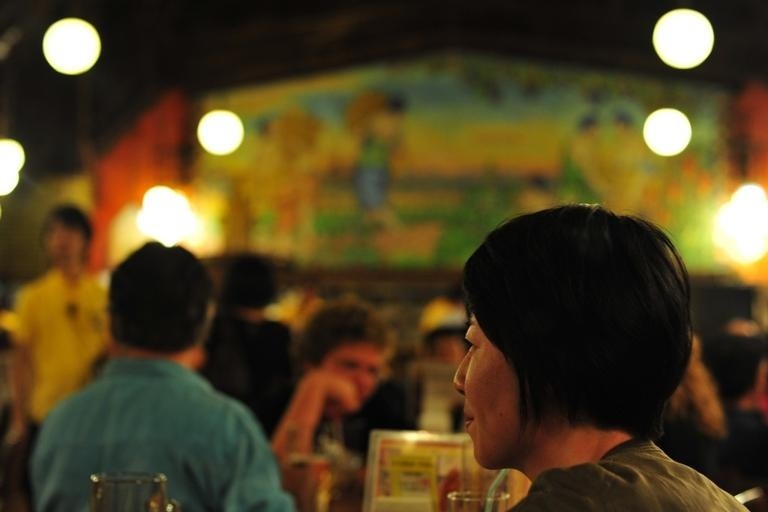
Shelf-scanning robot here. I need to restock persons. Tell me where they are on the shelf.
[265,300,406,467]
[670,327,732,438]
[200,252,298,440]
[29,242,297,512]
[689,314,767,491]
[454,201,750,511]
[6,201,110,445]
[422,293,470,362]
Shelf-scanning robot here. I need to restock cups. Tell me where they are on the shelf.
[447,491,510,512]
[287,453,332,512]
[89,471,168,511]
